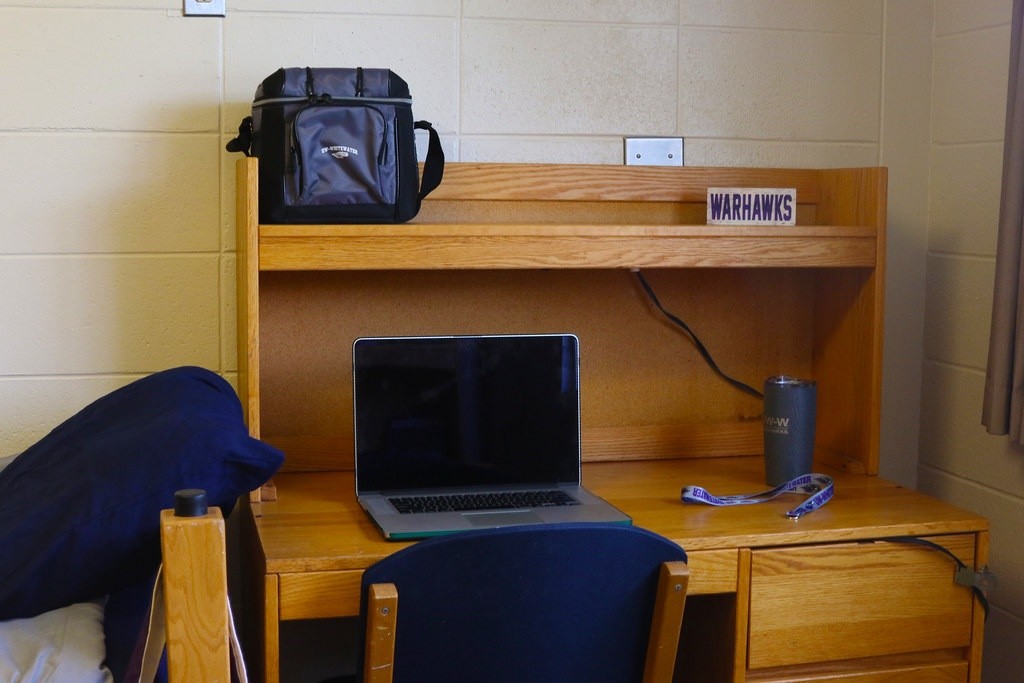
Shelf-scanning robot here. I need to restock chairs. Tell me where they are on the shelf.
[357,522,691,683]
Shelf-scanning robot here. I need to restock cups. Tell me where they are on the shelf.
[765,379,815,487]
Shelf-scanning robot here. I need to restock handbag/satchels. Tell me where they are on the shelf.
[226,67,445,224]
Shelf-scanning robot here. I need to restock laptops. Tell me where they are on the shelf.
[351,332,633,541]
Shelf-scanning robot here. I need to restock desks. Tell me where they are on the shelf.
[230,162,990,683]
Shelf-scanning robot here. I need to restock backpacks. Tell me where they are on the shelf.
[0,366,284,623]
